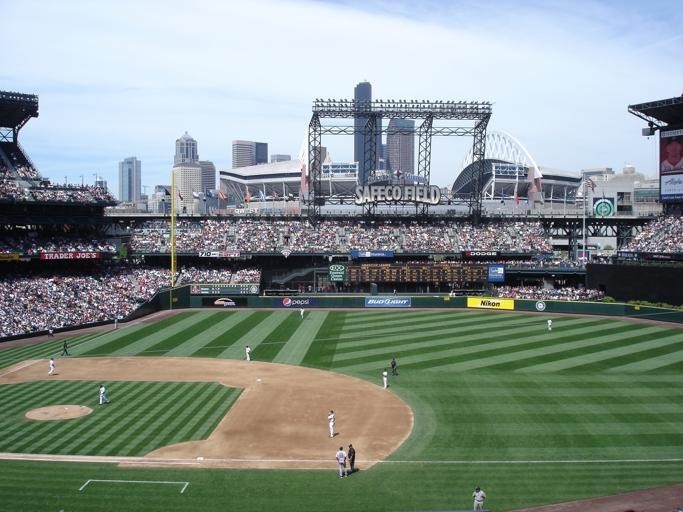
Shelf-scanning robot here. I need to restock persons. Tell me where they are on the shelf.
[98,385,110,405]
[659,137,682,171]
[244,345,251,361]
[381,368,388,389]
[48,357,56,376]
[335,446,347,479]
[327,410,335,437]
[390,358,397,376]
[471,486,486,512]
[347,444,356,472]
[60,339,70,357]
[0,152,682,340]
